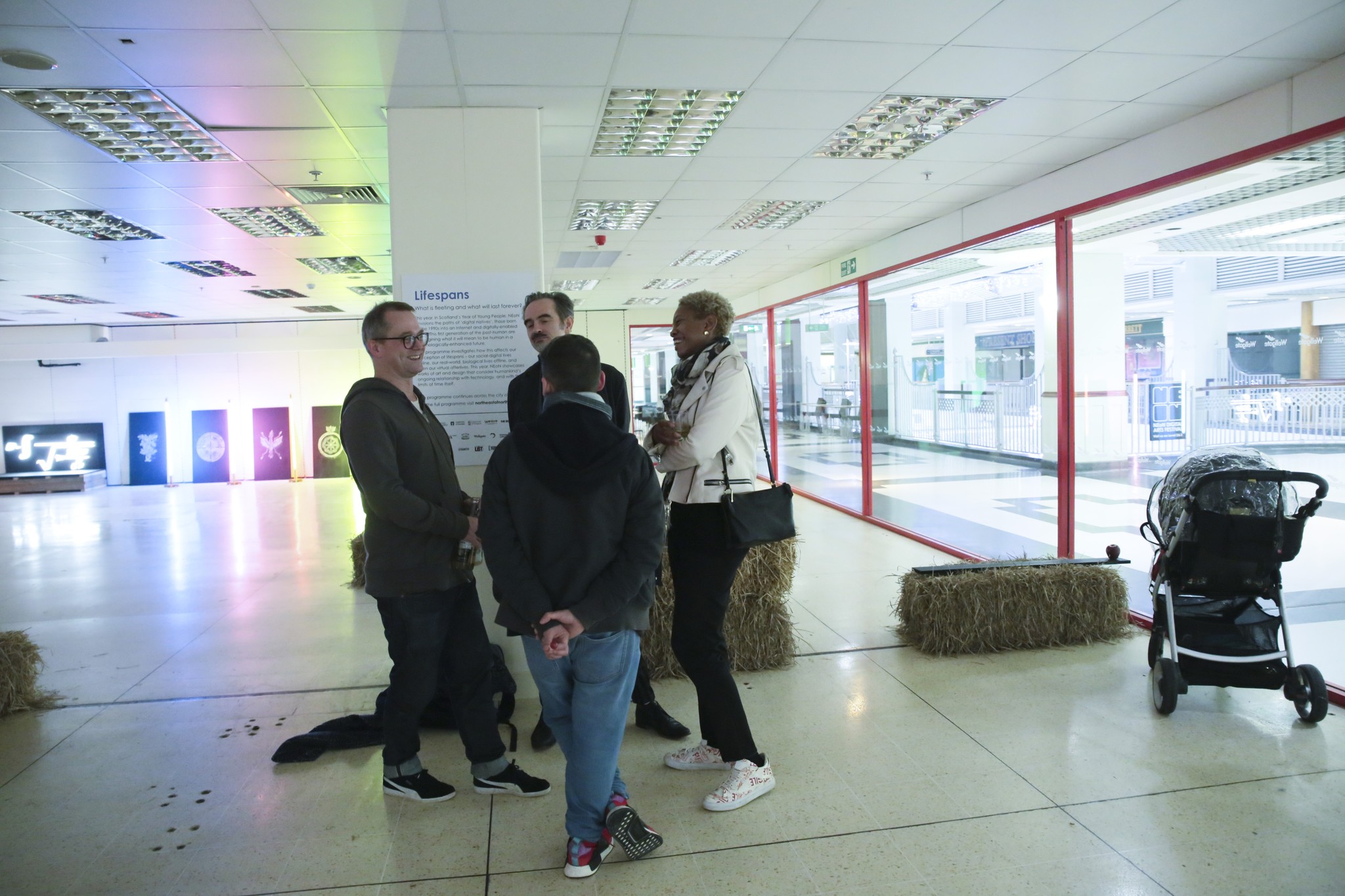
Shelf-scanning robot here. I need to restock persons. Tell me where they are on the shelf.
[644,289,777,812]
[474,334,664,879]
[507,291,691,753]
[341,302,552,803]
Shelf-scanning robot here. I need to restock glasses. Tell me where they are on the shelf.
[373,332,429,349]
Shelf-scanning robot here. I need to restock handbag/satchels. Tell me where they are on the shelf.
[720,480,796,549]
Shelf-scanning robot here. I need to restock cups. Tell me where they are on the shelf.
[663,409,691,441]
[453,497,483,571]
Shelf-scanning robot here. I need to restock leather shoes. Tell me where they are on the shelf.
[531,707,557,750]
[635,700,691,739]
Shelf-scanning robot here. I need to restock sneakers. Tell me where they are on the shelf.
[702,750,776,810]
[473,761,551,798]
[382,753,457,802]
[663,739,732,771]
[604,795,663,861]
[563,827,614,878]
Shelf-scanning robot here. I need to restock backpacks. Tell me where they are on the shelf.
[419,642,519,753]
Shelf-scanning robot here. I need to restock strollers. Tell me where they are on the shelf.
[1141,448,1327,721]
